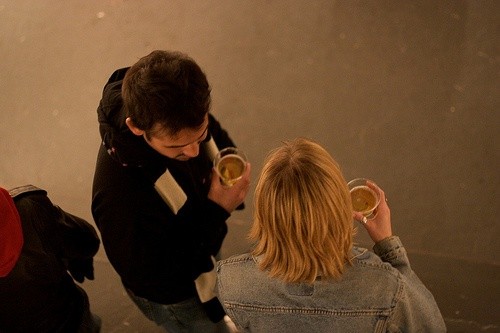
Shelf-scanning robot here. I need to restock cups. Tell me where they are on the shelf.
[214,148,248,186]
[347,178,381,218]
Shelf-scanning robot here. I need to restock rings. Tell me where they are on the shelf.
[360,216,368,225]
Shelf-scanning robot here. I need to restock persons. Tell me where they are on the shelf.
[0,184,101,333]
[90,49,250,333]
[216,137,450,333]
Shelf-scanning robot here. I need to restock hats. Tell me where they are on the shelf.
[0,187,24,279]
